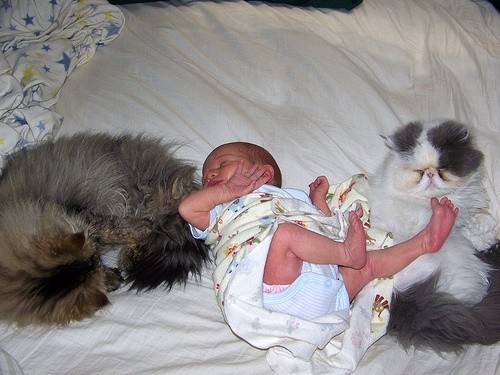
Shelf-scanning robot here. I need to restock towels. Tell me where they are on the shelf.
[202,173,396,375]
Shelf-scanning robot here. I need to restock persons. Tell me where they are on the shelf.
[178,141,459,362]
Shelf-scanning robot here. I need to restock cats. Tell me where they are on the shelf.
[1,128,213,331]
[374,118,500,361]
